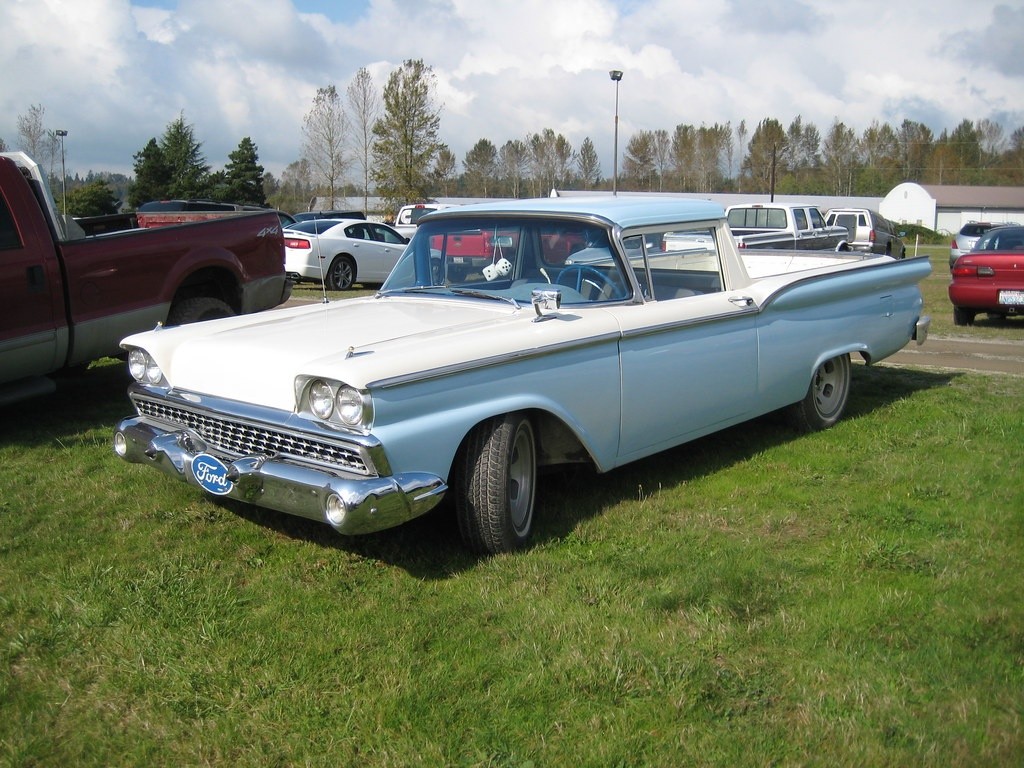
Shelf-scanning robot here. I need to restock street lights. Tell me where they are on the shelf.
[55,129,70,239]
[610,69,624,194]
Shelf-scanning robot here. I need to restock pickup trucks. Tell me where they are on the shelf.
[663,203,850,257]
[0,151,293,392]
[111,196,932,555]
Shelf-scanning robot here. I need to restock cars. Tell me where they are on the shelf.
[395,203,462,239]
[428,224,588,270]
[282,217,411,289]
[565,228,664,267]
[951,222,1002,269]
[949,223,1024,326]
[138,201,299,232]
[281,211,376,241]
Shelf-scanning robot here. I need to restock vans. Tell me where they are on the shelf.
[823,207,906,260]
